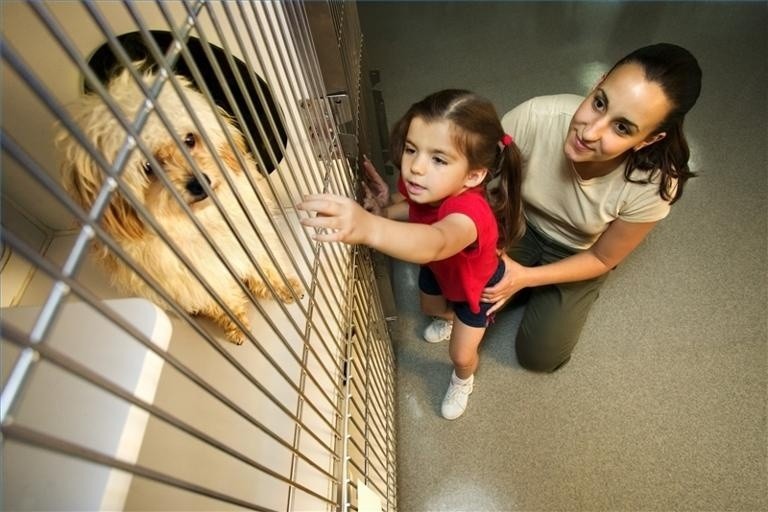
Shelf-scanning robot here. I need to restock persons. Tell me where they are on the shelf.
[295,89,523,422]
[478,40,704,376]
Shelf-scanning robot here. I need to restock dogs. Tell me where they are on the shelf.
[51,60,306,346]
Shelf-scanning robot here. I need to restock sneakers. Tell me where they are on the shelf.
[423,317,453,344]
[440,368,474,421]
